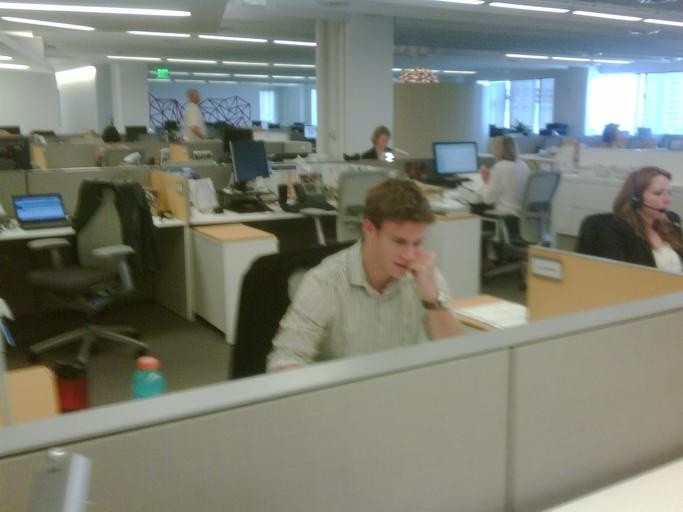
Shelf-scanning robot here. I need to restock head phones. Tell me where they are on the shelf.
[629,174,642,208]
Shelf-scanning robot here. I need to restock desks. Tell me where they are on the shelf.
[2,121,470,242]
[1,246,681,512]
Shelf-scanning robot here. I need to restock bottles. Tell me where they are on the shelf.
[50,356,89,415]
[127,354,164,402]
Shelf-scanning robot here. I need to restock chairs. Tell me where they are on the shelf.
[482,171,566,290]
[21,174,153,376]
[297,164,400,257]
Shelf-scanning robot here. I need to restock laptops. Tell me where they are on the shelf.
[10,193,73,231]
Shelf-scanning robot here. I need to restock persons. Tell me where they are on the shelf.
[183,89,206,142]
[95,126,130,166]
[594,167,683,275]
[472,136,529,261]
[361,125,393,159]
[265,179,462,374]
[601,124,623,148]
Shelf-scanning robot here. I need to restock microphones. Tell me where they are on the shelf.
[643,204,666,213]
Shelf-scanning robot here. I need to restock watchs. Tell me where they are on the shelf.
[421,289,449,310]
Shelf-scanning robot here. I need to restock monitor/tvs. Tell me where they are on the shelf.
[125,126,146,138]
[303,125,317,140]
[165,119,177,129]
[0,134,32,169]
[432,141,478,176]
[229,140,269,186]
[223,126,253,152]
